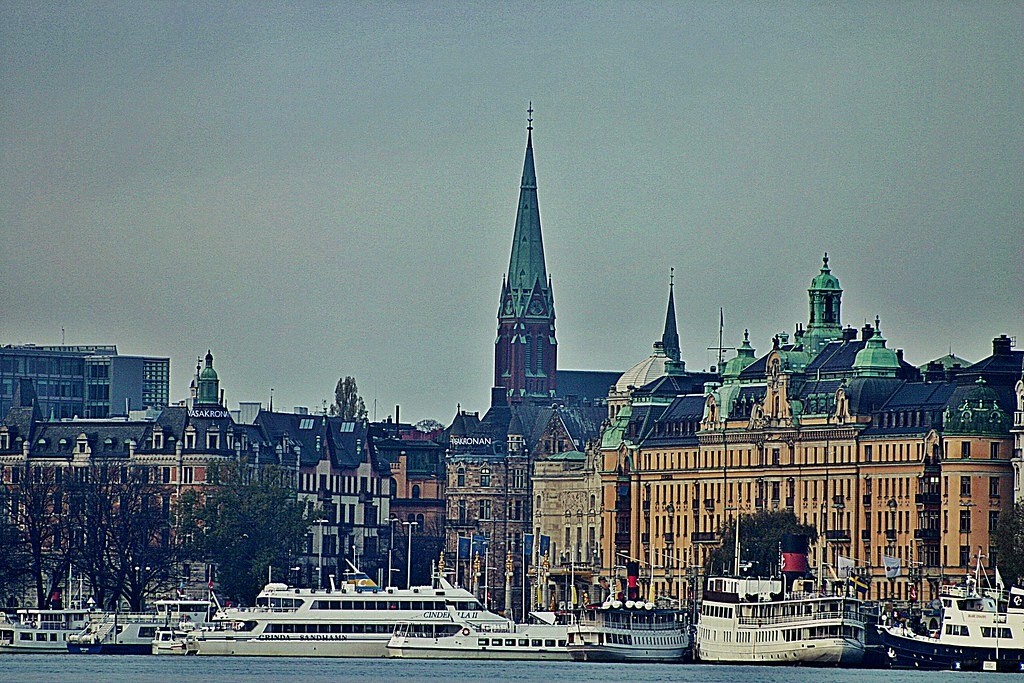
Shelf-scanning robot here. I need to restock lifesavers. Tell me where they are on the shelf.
[462,628,469,636]
[62,622,66,628]
[31,621,36,628]
[85,622,89,627]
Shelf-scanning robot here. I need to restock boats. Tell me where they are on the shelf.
[861,544,1024,675]
[694,491,870,669]
[0,563,110,655]
[66,593,247,656]
[563,544,696,664]
[386,530,580,662]
[151,549,515,660]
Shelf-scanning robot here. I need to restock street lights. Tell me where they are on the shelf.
[959,502,977,573]
[831,503,846,579]
[312,518,329,589]
[384,518,399,550]
[402,522,419,589]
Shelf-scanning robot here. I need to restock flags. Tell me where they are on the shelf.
[539,534,550,557]
[523,532,534,555]
[849,572,869,593]
[883,555,902,578]
[837,555,856,578]
[457,535,484,561]
[996,566,1005,590]
